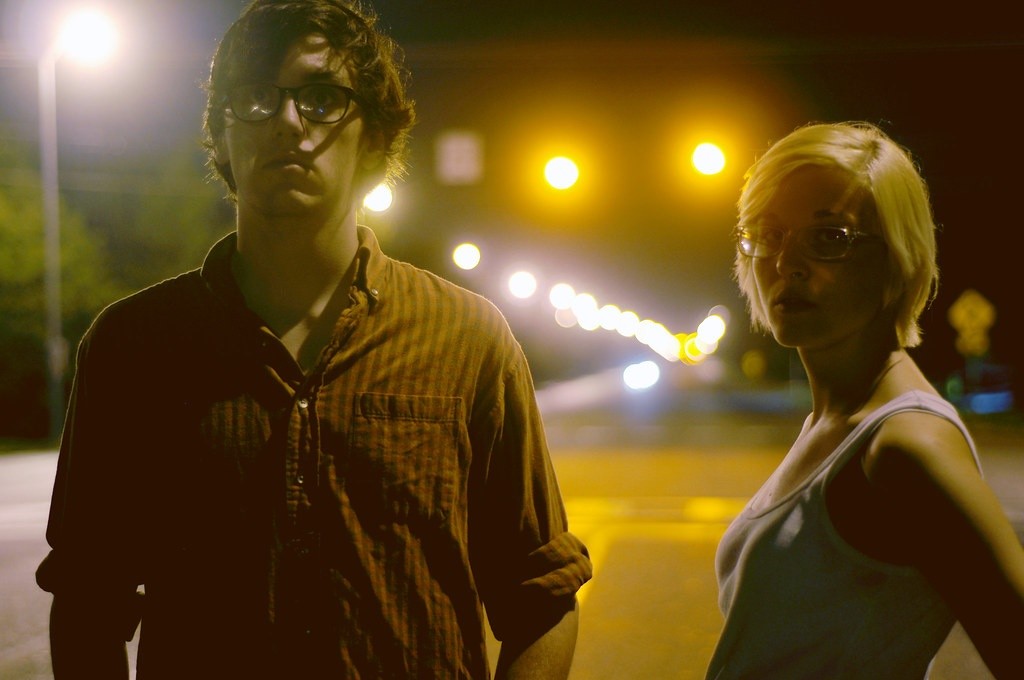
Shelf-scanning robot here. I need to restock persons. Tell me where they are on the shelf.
[705,122,1023,680]
[37,0,592,680]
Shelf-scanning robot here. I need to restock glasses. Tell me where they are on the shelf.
[735,223,886,259]
[218,79,373,123]
